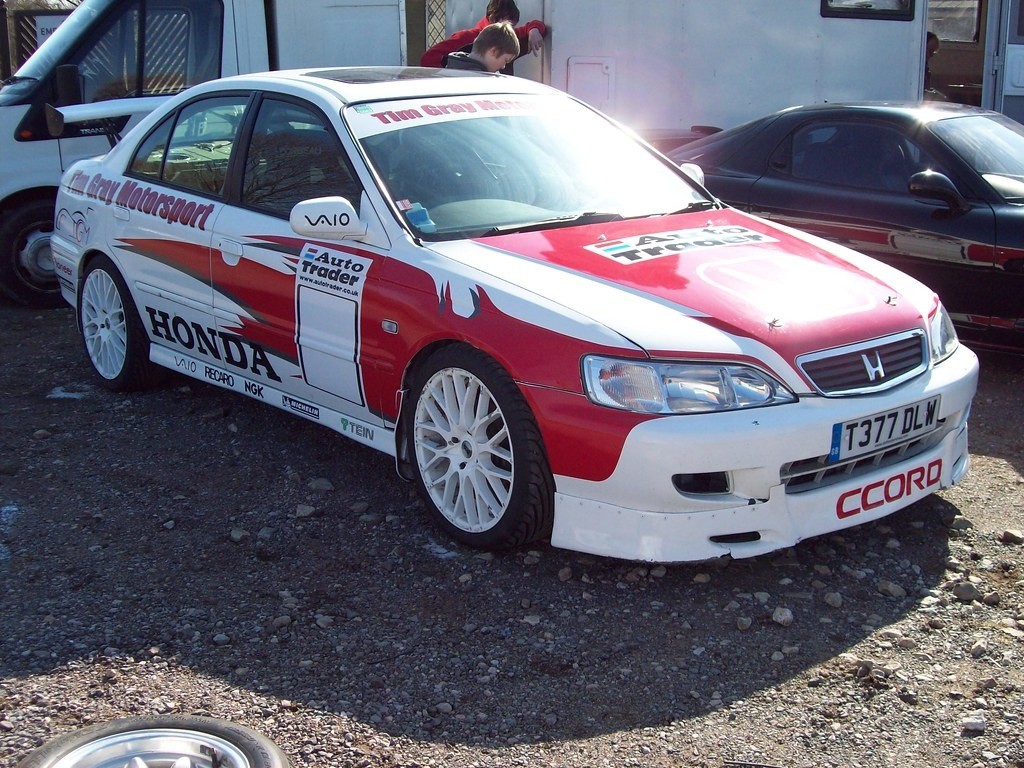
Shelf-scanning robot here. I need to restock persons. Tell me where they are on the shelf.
[445,21,520,75]
[923,30,944,102]
[419,0,546,78]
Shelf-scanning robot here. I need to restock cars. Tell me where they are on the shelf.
[658,97,1024,361]
[46,61,979,563]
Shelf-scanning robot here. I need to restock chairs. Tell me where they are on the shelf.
[257,129,358,207]
[877,140,913,193]
[396,148,486,200]
[805,143,850,183]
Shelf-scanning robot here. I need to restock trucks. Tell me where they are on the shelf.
[0,0,1024,317]
